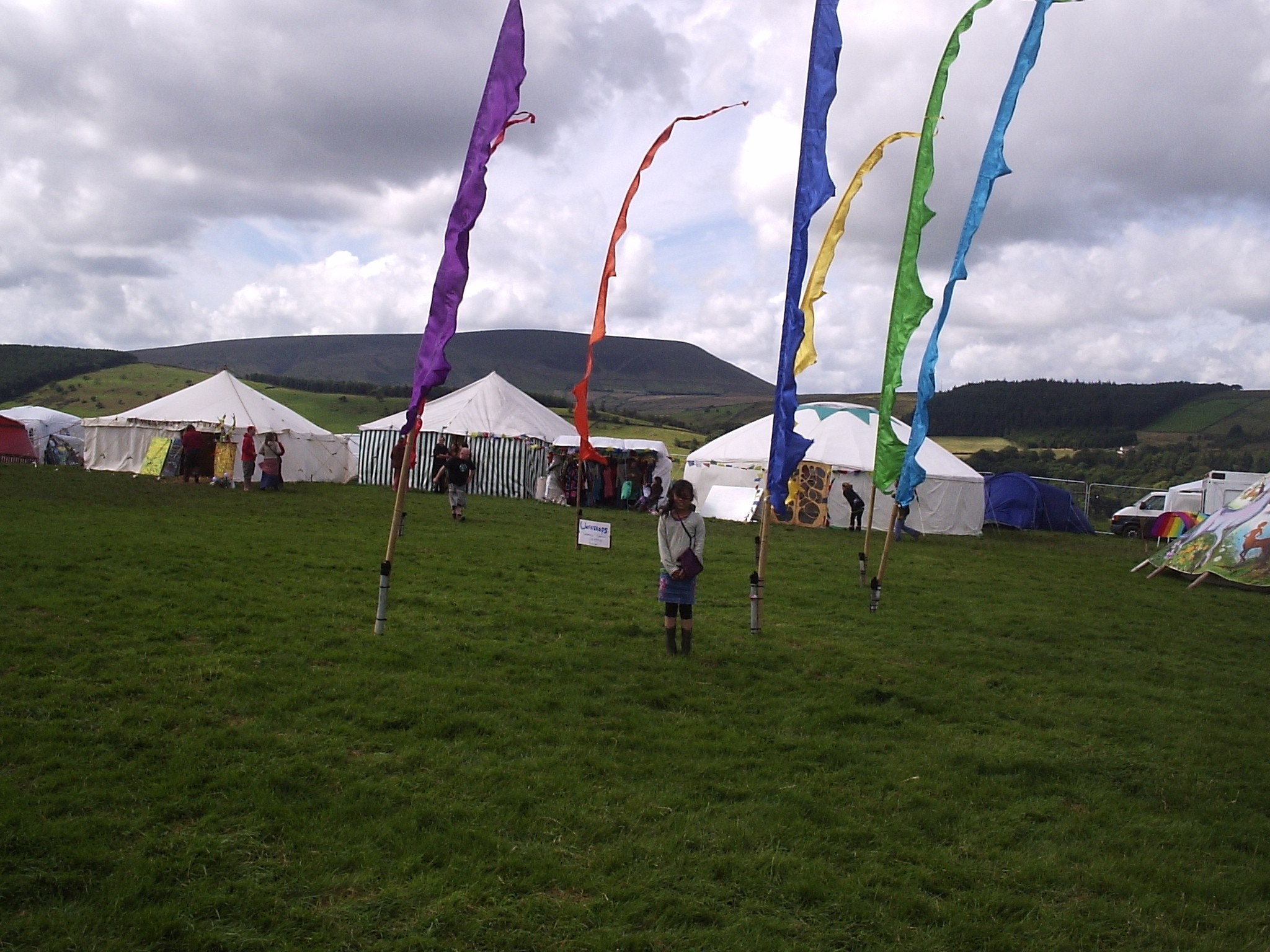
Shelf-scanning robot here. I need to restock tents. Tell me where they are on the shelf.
[985,473,1098,538]
[355,369,582,501]
[1131,470,1269,594]
[678,400,985,539]
[545,435,672,517]
[80,369,348,485]
[0,405,85,467]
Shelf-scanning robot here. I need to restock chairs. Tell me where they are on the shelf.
[645,497,664,513]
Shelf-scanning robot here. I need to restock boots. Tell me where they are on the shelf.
[628,501,644,512]
[665,626,678,655]
[681,626,693,654]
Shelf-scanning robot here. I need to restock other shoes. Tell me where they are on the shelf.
[913,533,923,543]
[244,487,253,492]
[849,526,855,531]
[856,526,862,531]
[451,510,466,522]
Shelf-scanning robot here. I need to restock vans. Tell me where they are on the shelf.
[1197,470,1270,519]
[1110,491,1208,543]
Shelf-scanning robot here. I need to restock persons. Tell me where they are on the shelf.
[627,460,641,491]
[241,426,258,491]
[657,479,705,658]
[840,482,865,531]
[258,432,286,490]
[627,476,663,512]
[180,425,205,485]
[433,436,472,493]
[432,448,475,521]
[893,478,921,543]
[390,437,405,491]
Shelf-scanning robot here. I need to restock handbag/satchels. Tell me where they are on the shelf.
[454,473,467,486]
[677,547,704,580]
[643,485,651,498]
[853,502,863,511]
